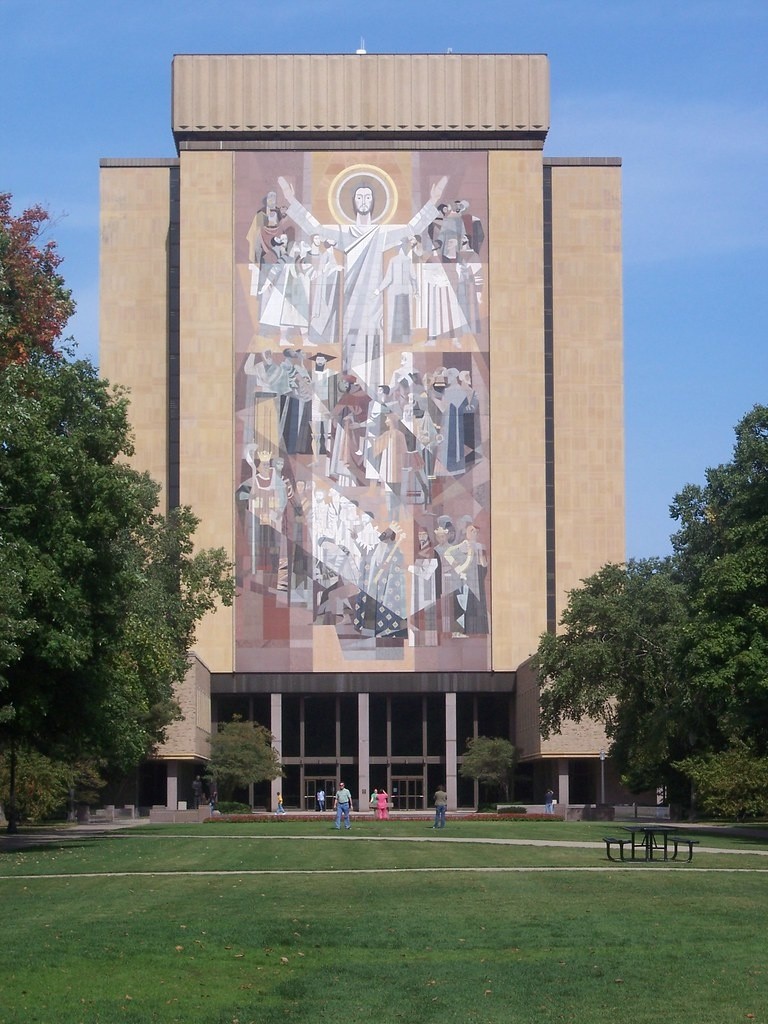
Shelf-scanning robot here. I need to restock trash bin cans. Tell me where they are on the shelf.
[589,804,617,821]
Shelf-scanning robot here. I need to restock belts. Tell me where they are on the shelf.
[338,802,348,804]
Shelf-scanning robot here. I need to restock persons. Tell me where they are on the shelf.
[376,789,388,820]
[317,787,326,812]
[370,789,378,811]
[192,776,201,809]
[274,792,286,815]
[334,782,353,829]
[433,785,448,828]
[545,788,555,813]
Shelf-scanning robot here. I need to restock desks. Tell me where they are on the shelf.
[625,827,679,862]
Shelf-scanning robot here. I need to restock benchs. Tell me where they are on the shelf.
[602,837,632,862]
[667,836,700,863]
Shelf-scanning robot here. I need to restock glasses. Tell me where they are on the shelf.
[340,785,344,786]
